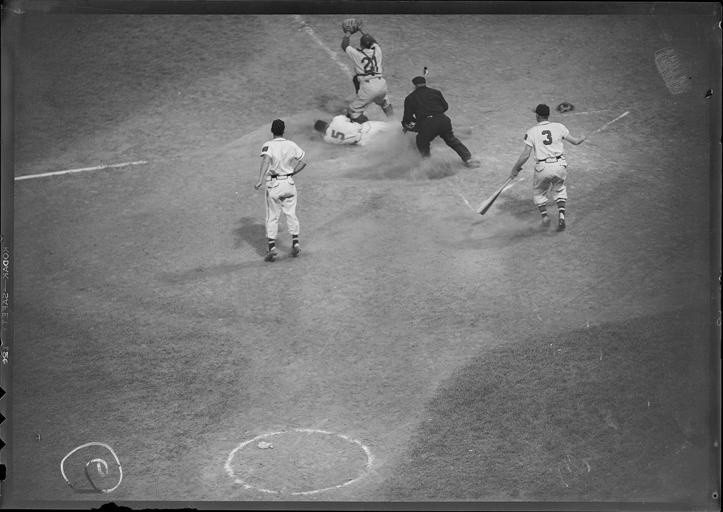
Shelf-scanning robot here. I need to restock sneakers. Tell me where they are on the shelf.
[466,159,480,167]
[559,213,565,230]
[264,248,280,262]
[293,243,301,257]
[544,217,550,228]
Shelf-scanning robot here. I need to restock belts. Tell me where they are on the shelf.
[538,156,560,162]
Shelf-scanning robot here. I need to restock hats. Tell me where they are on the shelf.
[535,104,550,115]
[272,174,294,178]
[271,119,284,134]
[412,76,426,85]
[314,120,328,131]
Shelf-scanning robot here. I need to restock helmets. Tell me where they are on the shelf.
[360,35,374,48]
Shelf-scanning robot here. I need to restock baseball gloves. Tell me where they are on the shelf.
[343,17,362,33]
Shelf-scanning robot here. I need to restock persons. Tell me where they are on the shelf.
[341,19,395,124]
[315,114,389,146]
[510,104,586,229]
[254,119,308,262]
[401,76,474,165]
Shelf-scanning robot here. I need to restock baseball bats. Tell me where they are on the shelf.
[480,176,512,214]
